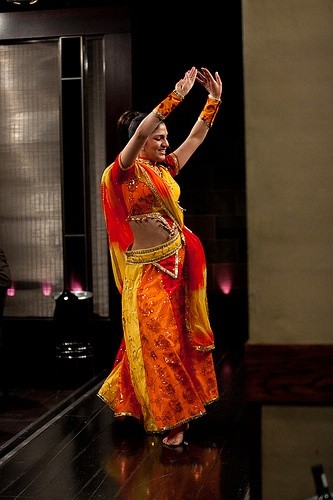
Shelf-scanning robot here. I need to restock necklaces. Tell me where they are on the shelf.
[139,159,173,192]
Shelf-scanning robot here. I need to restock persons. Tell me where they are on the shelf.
[96,65,223,446]
